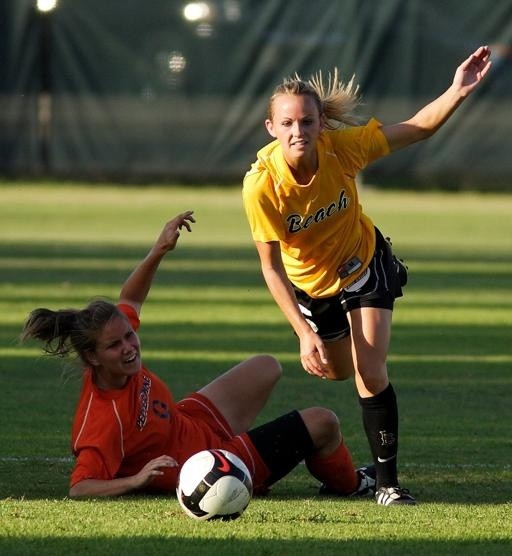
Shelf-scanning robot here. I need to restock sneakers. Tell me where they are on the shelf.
[318,465,376,497]
[375,483,417,506]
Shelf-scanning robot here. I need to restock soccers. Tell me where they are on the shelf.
[178,448,254,522]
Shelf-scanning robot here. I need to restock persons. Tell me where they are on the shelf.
[238,40,493,508]
[19,208,377,503]
